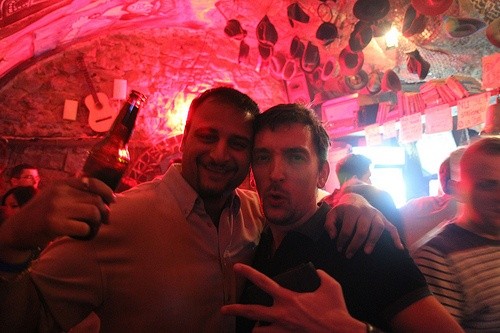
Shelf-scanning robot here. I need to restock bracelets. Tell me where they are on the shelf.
[366,323,374,333]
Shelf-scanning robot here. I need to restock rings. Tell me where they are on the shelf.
[81,176,90,189]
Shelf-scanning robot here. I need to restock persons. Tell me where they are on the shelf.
[219,101,464,333]
[0,87,406,333]
[0,163,44,280]
[316,153,463,252]
[397,138,500,333]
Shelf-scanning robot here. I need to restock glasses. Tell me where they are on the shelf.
[16,175,41,183]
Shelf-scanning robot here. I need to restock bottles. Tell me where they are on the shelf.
[68,90,147,240]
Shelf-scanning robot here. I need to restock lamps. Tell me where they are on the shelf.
[112,79,127,110]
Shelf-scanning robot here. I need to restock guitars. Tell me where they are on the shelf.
[75,50,117,133]
